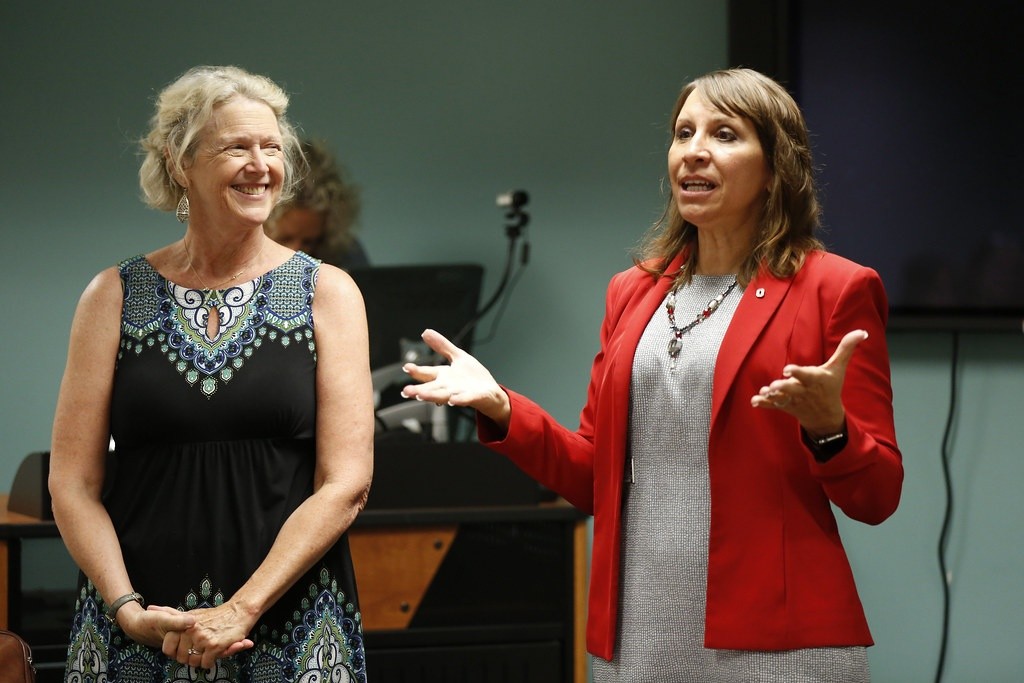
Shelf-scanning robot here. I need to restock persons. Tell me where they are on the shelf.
[264,141,374,312]
[402,67,906,683]
[47,66,376,683]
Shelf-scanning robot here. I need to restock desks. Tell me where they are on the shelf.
[0,492,590,683]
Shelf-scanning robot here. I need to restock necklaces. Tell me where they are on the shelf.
[666,261,740,358]
[183,236,267,291]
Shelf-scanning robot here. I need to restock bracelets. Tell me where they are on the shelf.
[105,592,144,623]
[801,421,847,448]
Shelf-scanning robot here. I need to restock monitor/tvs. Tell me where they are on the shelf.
[349,265,484,395]
[777,0,1024,330]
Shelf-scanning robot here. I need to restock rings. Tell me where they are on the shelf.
[188,647,201,655]
